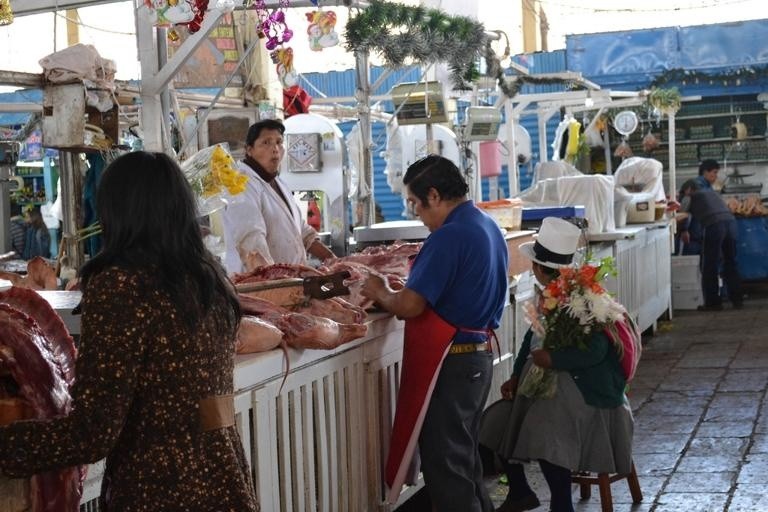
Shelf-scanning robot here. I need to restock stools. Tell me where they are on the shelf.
[570,456,643,511]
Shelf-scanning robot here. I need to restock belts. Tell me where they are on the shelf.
[449,341,488,354]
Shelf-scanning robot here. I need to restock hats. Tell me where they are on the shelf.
[680,179,704,195]
[518,217,580,270]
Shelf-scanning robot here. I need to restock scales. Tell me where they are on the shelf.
[723,108,756,177]
[462,106,541,227]
[521,205,586,230]
[614,110,638,163]
[353,82,450,241]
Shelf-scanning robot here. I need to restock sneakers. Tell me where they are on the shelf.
[697,300,744,310]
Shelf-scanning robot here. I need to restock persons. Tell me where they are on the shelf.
[0,151,260,511]
[360,152,510,512]
[221,120,339,278]
[476,217,642,511]
[675,159,749,301]
[678,180,745,311]
[22,206,51,259]
[9,199,26,257]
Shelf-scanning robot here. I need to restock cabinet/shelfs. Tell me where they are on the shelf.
[635,110,768,169]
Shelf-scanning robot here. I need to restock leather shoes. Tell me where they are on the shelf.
[495,494,540,511]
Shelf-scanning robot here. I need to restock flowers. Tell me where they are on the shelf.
[520,244,628,398]
